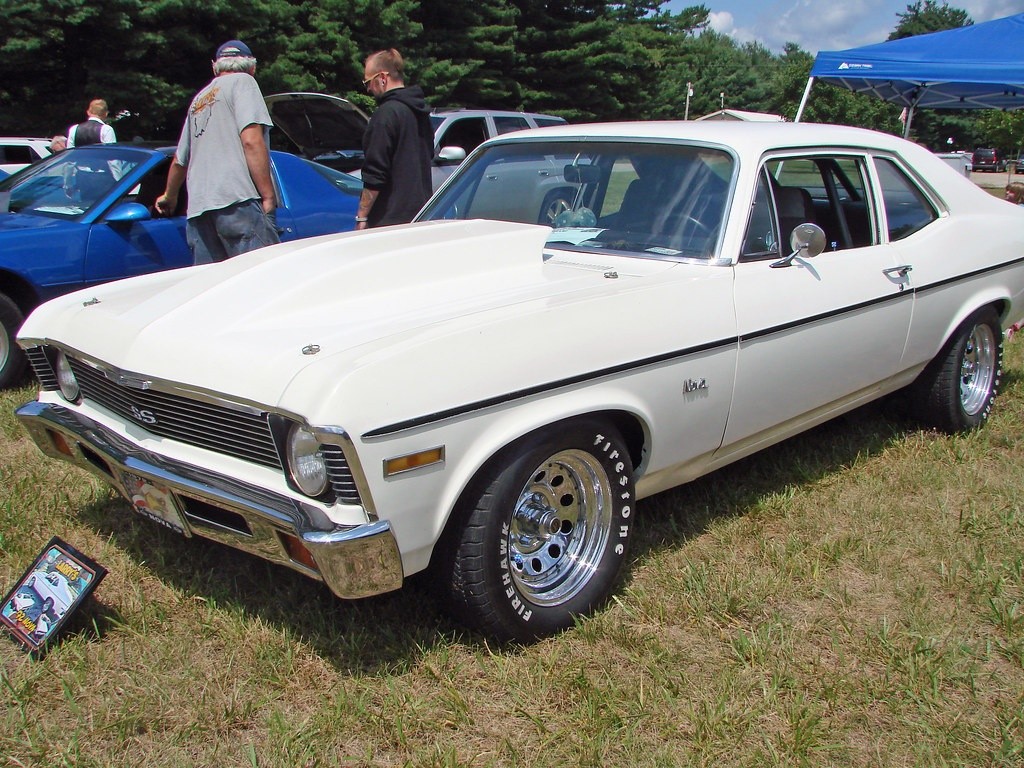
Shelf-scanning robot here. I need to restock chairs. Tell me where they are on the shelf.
[758,186,815,254]
[608,180,676,235]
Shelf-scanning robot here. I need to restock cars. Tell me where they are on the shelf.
[0,142,371,390]
[0,137,80,200]
[261,91,591,228]
[1015,154,1024,174]
[11,117,1024,651]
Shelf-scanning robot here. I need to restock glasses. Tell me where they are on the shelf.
[362,71,388,88]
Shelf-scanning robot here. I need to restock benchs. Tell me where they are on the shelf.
[682,192,923,249]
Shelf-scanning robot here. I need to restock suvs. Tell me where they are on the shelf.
[971,147,1004,175]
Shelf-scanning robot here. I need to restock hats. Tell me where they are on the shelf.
[216,40,252,58]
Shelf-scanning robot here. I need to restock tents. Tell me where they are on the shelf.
[769,13,1024,183]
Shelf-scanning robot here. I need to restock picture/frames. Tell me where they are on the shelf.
[0,536,109,661]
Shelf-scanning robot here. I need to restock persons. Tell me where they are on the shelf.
[62,100,123,205]
[154,40,277,266]
[353,47,439,231]
[49,136,69,154]
[586,145,739,262]
[1004,184,1023,207]
